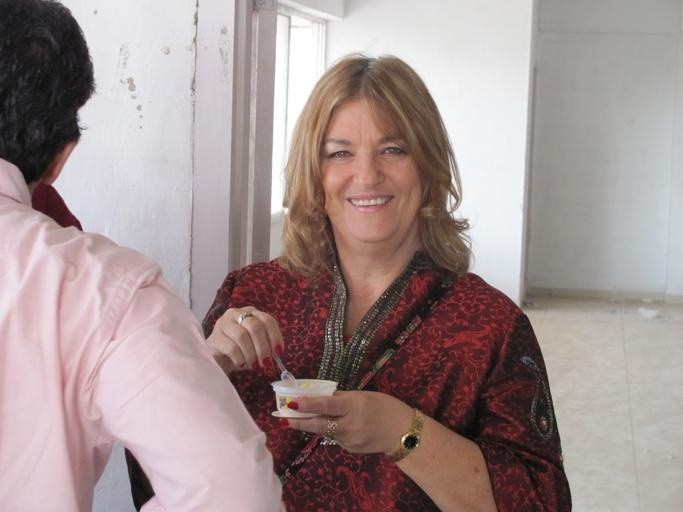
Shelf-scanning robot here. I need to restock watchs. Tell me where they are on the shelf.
[389,408,424,462]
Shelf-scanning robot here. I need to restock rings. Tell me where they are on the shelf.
[319,437,337,446]
[328,417,337,434]
[239,311,253,323]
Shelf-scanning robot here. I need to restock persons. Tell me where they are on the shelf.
[0,2,282,512]
[126,49,571,512]
